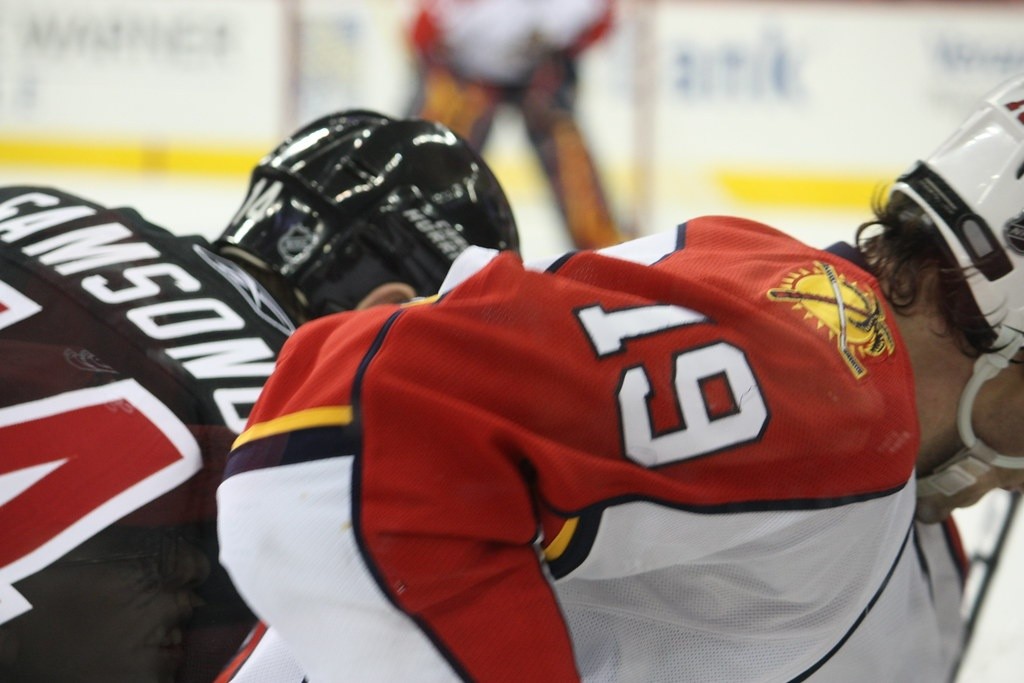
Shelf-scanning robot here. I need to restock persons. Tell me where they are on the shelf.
[390,2,621,253]
[215,73,1024,683]
[0,109,521,683]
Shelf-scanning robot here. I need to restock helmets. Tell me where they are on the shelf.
[212,109,522,317]
[893,73,1024,367]
